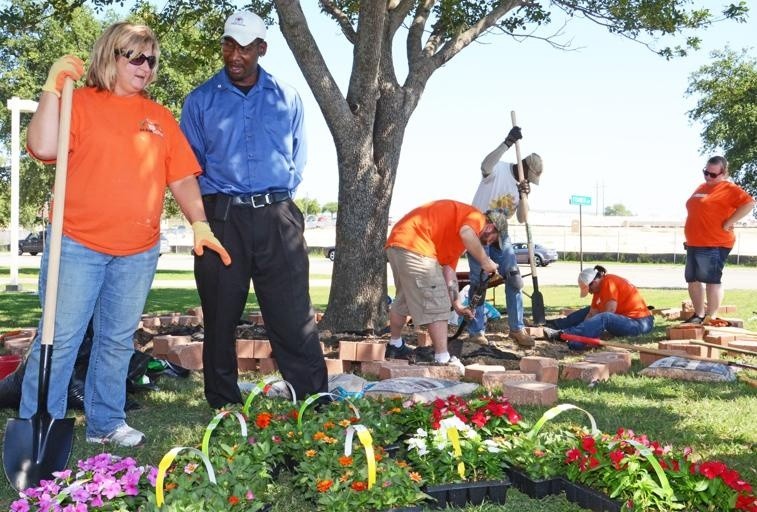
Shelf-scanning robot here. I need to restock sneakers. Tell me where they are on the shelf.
[542,328,566,341]
[510,327,535,347]
[468,332,488,345]
[386,342,414,360]
[85,422,146,447]
[684,315,705,323]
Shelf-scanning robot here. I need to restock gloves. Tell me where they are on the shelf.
[192,222,231,267]
[504,126,522,148]
[516,180,530,199]
[42,54,84,97]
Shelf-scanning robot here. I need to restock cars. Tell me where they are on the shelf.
[159,232,171,258]
[734,219,752,226]
[5,229,46,257]
[510,240,559,268]
[304,208,393,229]
[321,245,336,262]
[166,223,186,234]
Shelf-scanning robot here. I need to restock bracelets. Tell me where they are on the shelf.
[505,137,516,143]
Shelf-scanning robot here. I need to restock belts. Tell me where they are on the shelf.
[203,190,289,209]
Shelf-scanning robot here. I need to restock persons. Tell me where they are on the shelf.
[542,264,655,350]
[465,125,543,349]
[179,10,331,430]
[682,155,755,325]
[382,197,507,365]
[19,20,232,449]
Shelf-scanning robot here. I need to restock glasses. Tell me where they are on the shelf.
[217,43,262,55]
[114,46,156,70]
[703,166,724,178]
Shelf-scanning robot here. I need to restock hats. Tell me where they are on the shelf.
[579,269,599,296]
[525,153,542,185]
[219,12,266,46]
[487,210,508,250]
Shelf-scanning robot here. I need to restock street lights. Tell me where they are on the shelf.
[5,96,38,292]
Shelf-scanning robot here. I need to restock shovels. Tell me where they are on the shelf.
[3,76,76,500]
[510,111,546,326]
[448,295,481,358]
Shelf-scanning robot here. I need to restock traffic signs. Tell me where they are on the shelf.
[570,193,593,207]
